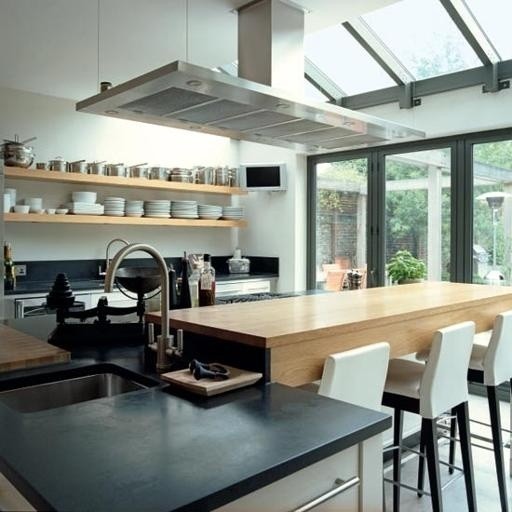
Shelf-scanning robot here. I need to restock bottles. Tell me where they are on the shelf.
[4,242,16,290]
[168,250,216,307]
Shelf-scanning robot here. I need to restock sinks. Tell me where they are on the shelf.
[0,359,172,416]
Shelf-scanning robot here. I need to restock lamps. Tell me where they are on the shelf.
[477,188,510,268]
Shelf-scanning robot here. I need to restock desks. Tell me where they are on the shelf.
[145,281,512,395]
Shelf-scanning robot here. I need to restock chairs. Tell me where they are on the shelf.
[315,341,391,511]
[381,321,476,511]
[417,308,511,511]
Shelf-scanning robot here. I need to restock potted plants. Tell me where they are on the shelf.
[386,249,427,284]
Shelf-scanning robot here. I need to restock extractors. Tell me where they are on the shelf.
[75,1,427,156]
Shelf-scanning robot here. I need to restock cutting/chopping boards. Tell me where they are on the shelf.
[0,313,71,371]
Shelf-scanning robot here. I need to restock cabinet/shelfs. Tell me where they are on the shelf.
[2,162,252,230]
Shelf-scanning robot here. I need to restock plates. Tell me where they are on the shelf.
[48,209,56,214]
[103,195,244,218]
[56,209,69,215]
[72,191,104,215]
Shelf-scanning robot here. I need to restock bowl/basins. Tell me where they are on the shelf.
[1,135,37,168]
[49,159,240,188]
[3,186,44,215]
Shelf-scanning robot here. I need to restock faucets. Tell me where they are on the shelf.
[97,237,132,278]
[103,242,185,370]
